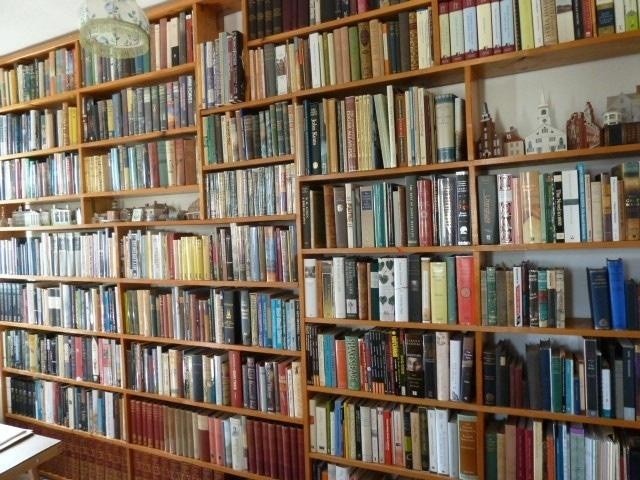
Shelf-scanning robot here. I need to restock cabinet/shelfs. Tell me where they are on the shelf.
[1,2,639,480]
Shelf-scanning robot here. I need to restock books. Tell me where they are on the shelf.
[483,414,628,480]
[0,223,296,282]
[302,255,474,326]
[0,48,75,107]
[0,329,121,387]
[481,335,640,421]
[126,341,303,418]
[302,172,470,248]
[247,1,434,101]
[196,31,244,108]
[122,286,301,351]
[6,377,304,480]
[309,396,478,479]
[475,163,639,244]
[480,259,640,330]
[0,282,120,335]
[201,84,464,218]
[439,1,639,63]
[83,139,196,193]
[304,324,475,403]
[80,74,195,140]
[0,103,79,156]
[0,153,79,201]
[80,12,193,86]
